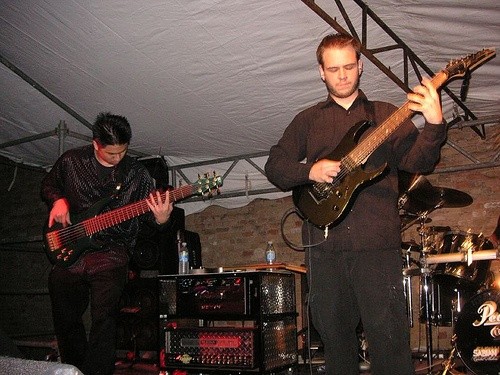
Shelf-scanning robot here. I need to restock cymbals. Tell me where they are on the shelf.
[394,170,474,225]
[418,226,451,235]
[402,240,437,254]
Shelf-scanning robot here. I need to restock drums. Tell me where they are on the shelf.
[452,286,500,375]
[421,232,494,285]
[417,273,495,327]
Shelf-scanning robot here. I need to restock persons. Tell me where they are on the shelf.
[266,33,447,375]
[41,115,175,375]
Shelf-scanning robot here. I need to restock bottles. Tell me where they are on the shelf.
[178,242,190,274]
[265,241,276,273]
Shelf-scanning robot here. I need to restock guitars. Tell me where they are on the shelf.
[41,170,223,266]
[292,48,496,229]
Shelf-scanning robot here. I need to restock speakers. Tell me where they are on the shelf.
[116,205,185,351]
